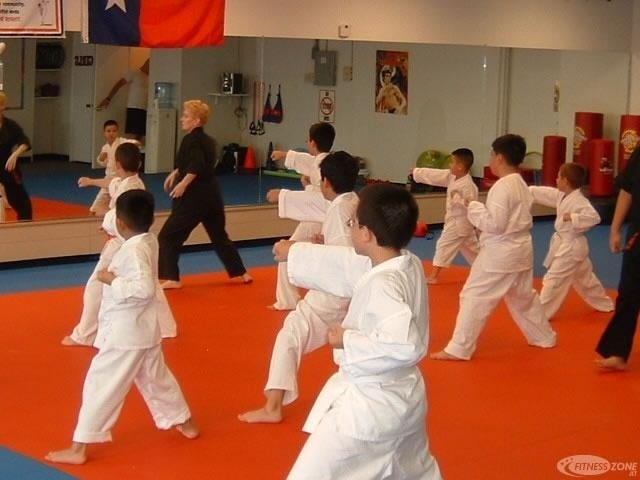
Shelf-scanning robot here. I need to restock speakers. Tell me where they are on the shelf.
[221,71,242,94]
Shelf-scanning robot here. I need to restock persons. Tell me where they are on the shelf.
[428,133,558,362]
[158,98,253,291]
[88,117,142,216]
[44,190,199,461]
[59,142,183,347]
[270,122,336,312]
[523,162,615,320]
[236,150,374,418]
[374,70,407,113]
[96,55,149,137]
[273,182,443,478]
[592,132,639,373]
[406,147,480,285]
[0,91,34,219]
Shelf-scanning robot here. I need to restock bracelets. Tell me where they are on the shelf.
[105,97,110,103]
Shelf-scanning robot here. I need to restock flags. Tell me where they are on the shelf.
[80,0,227,48]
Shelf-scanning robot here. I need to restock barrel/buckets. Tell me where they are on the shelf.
[354,170,369,185]
[154,82,172,108]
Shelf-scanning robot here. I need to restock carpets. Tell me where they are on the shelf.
[1,258,640,480]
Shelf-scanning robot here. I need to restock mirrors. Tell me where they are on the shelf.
[0,30,630,228]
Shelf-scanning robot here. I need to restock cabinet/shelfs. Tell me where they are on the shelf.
[34,68,62,102]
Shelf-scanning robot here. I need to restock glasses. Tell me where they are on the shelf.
[345,219,366,227]
